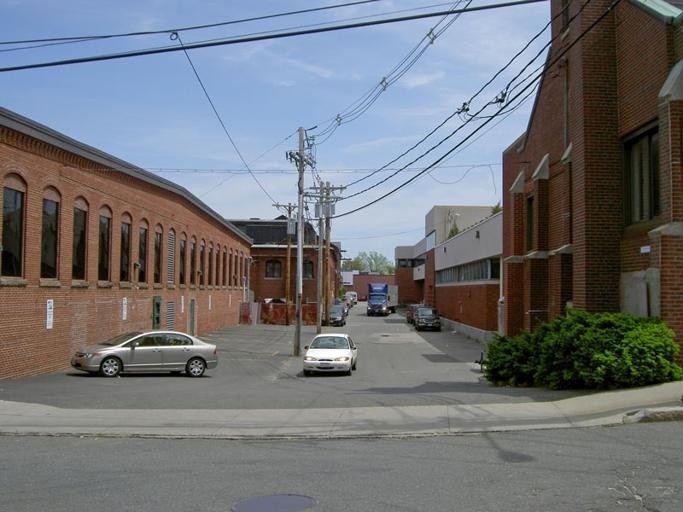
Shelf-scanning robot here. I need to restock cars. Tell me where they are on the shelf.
[406,302,441,333]
[72,328,218,382]
[304,332,359,378]
[320,292,358,326]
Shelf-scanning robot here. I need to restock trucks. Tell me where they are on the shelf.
[366,282,399,316]
